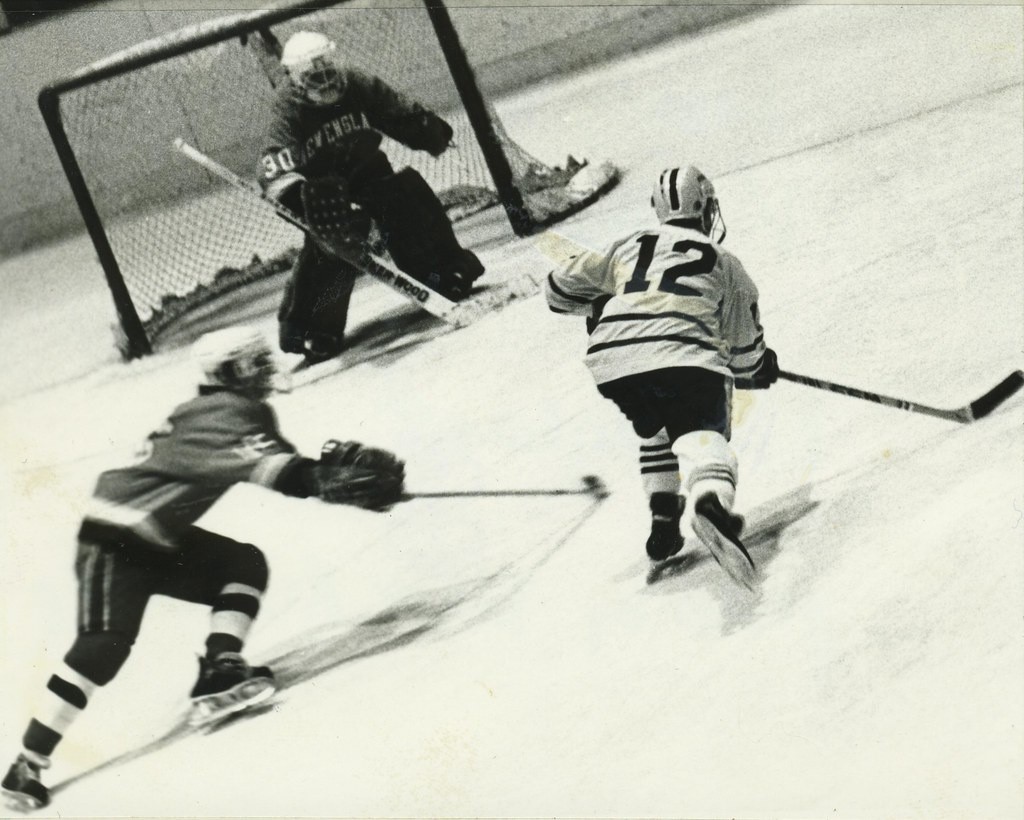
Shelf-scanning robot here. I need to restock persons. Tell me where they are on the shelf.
[256,29,540,372]
[545,164,779,601]
[0,340,406,814]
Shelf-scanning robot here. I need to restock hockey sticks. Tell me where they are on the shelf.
[401,472,611,503]
[774,365,1024,426]
[169,135,543,331]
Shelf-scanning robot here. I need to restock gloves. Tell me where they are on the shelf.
[318,439,404,513]
[734,347,778,390]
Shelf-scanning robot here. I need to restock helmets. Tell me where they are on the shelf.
[279,31,337,90]
[192,324,271,388]
[651,165,714,223]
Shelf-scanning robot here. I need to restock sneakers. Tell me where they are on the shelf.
[186,655,280,735]
[646,491,688,582]
[690,492,755,580]
[0,758,49,810]
